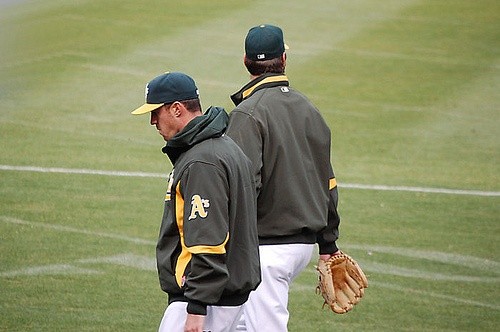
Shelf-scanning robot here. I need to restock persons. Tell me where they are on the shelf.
[129,70,263,332]
[224,22,367,331]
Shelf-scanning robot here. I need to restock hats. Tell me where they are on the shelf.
[245,24,290,61]
[131,72,199,115]
